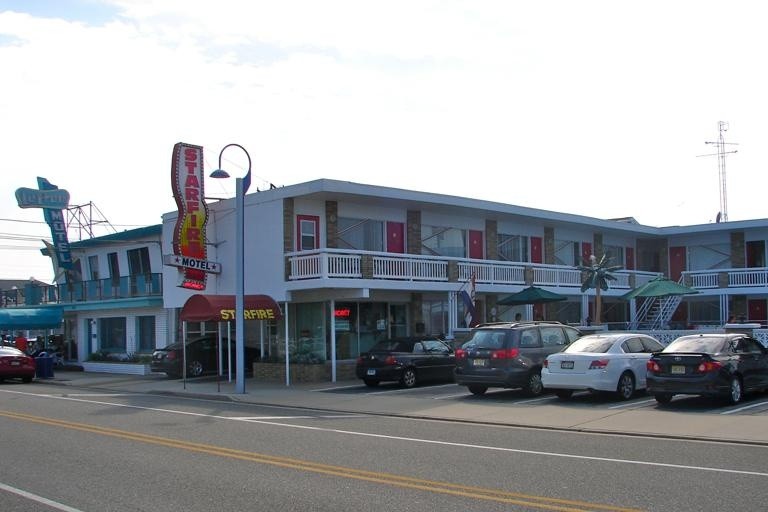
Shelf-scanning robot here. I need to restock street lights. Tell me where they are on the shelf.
[210,144,251,394]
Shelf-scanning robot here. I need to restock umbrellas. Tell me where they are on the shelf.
[615,273,700,330]
[495,284,567,321]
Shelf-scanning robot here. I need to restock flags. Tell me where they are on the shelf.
[459,273,477,328]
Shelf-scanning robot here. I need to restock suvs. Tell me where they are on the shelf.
[454,321,586,398]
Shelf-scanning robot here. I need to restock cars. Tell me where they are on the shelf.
[151,336,270,378]
[0,346,36,383]
[540,332,667,402]
[644,333,767,407]
[354,334,457,389]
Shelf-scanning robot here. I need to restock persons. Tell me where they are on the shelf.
[535,312,544,324]
[0,331,78,361]
[514,312,521,322]
[726,315,738,325]
